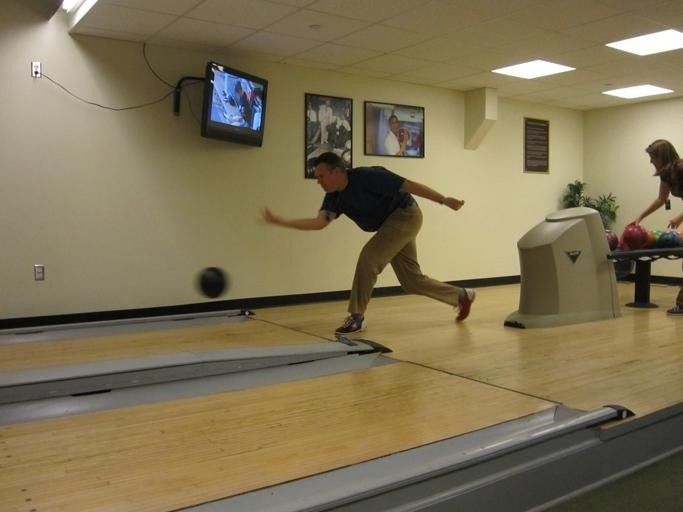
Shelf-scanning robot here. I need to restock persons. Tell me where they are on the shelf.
[260,151,476,334]
[318,99,332,143]
[232,81,252,126]
[627,140,683,316]
[383,115,408,155]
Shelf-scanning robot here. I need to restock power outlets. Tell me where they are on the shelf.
[32,62,42,79]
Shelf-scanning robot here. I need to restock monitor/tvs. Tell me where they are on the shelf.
[200,60,268,147]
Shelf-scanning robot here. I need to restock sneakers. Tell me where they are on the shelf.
[456,288,474,320]
[335,317,367,335]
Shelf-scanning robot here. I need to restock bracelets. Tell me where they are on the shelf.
[440,196,446,204]
